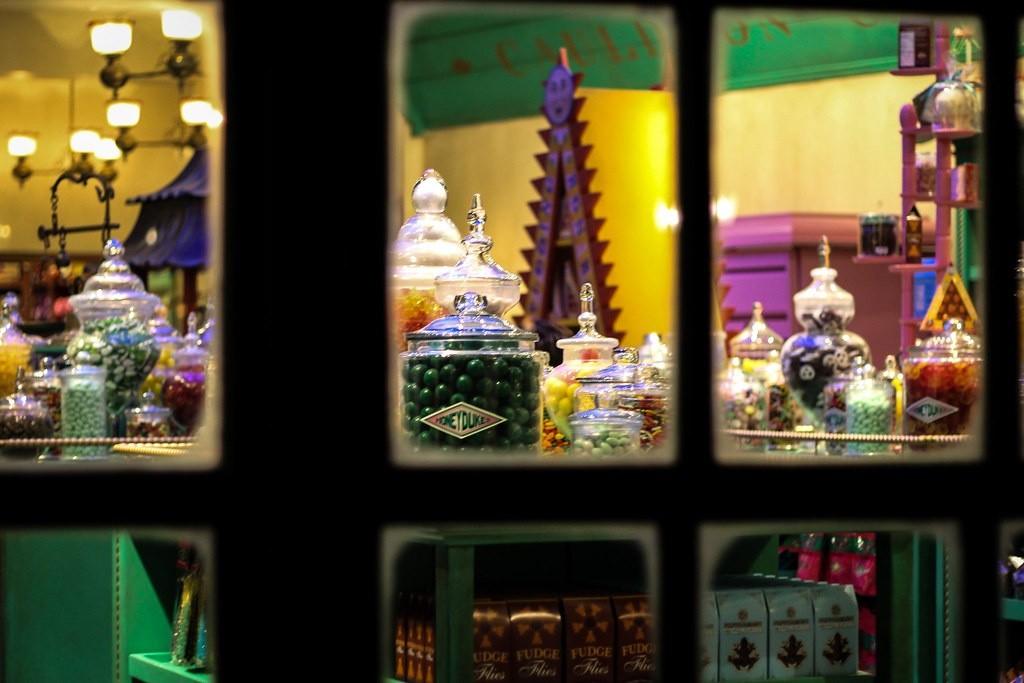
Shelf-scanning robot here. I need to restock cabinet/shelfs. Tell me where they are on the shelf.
[889,104,983,369]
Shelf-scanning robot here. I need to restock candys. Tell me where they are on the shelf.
[400,340,669,456]
[723,381,896,454]
[0,315,204,458]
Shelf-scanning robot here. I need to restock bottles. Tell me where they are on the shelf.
[914,150,936,197]
[906,203,922,264]
[711,236,980,453]
[858,212,898,256]
[392,170,676,458]
[0,239,215,460]
